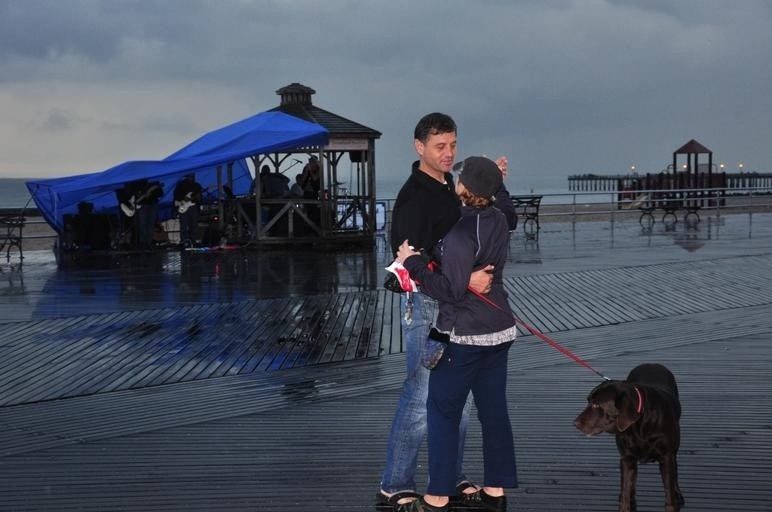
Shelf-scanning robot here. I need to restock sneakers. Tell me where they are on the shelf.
[395,497,450,511]
[461,488,507,511]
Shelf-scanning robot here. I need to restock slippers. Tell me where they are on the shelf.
[376,490,420,505]
[456,481,478,494]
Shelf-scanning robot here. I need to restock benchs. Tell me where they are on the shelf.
[0,213,26,263]
[638,192,704,223]
[508,195,543,234]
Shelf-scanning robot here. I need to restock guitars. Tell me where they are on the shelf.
[120,185,156,218]
[153,212,167,243]
[175,184,215,214]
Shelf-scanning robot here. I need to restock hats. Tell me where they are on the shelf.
[453,157,503,196]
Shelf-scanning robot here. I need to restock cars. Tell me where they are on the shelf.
[161,202,220,245]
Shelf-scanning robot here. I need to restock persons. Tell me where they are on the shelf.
[110,152,325,250]
[373,110,512,512]
[393,155,519,512]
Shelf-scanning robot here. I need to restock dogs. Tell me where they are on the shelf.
[573,362,686,512]
